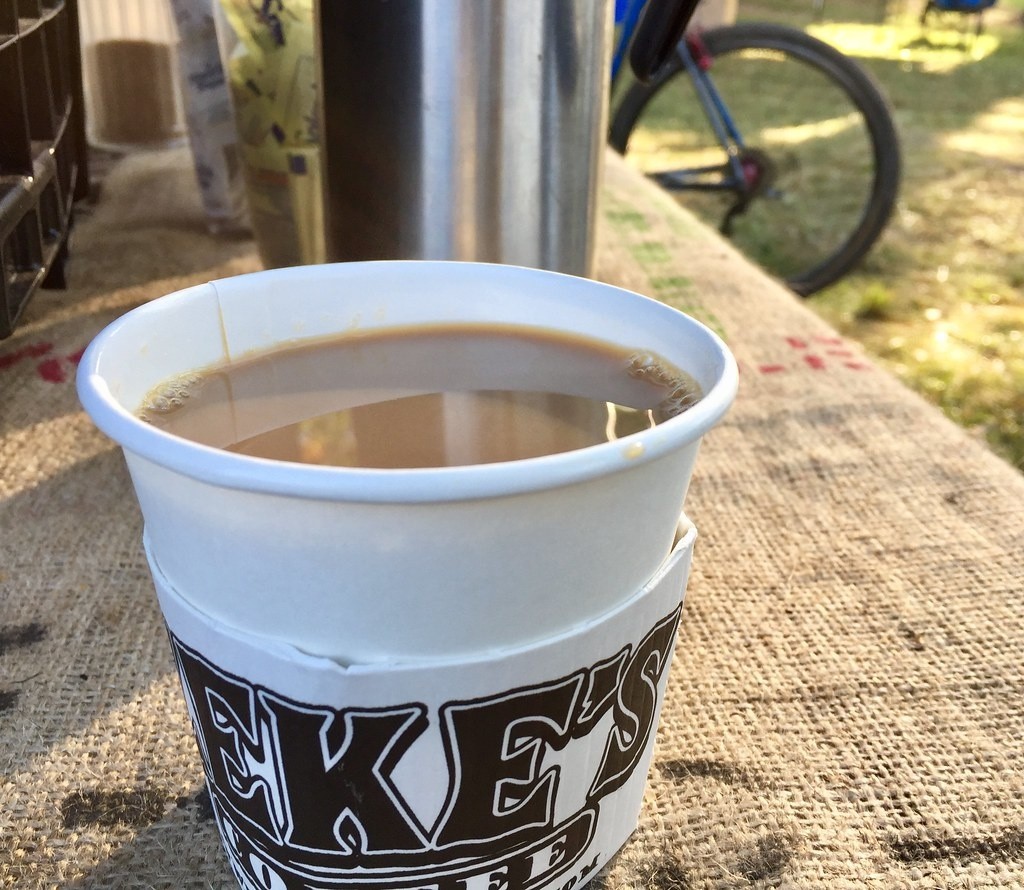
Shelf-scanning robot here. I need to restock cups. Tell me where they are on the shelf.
[74,0,191,151]
[210,0,330,266]
[77,259,740,890]
[165,1,256,242]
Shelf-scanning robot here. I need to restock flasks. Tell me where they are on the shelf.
[313,1,609,281]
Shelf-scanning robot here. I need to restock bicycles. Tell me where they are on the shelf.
[607,0,903,301]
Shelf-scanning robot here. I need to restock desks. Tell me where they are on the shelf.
[0,143,1024,889]
